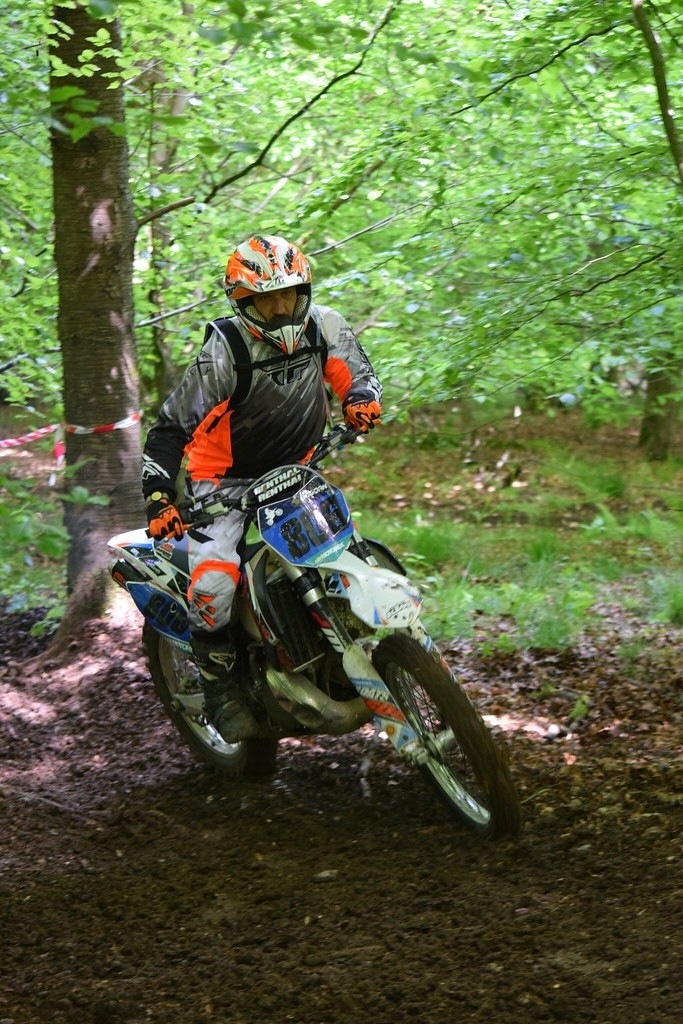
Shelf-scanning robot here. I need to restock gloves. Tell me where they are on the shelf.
[146,492,185,541]
[342,390,382,433]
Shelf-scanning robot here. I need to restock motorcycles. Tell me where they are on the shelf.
[107,421,530,847]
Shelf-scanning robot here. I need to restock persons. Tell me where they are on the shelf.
[136,233,381,740]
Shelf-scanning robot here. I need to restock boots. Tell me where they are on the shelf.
[188,617,261,744]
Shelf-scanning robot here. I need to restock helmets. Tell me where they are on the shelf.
[223,234,312,355]
